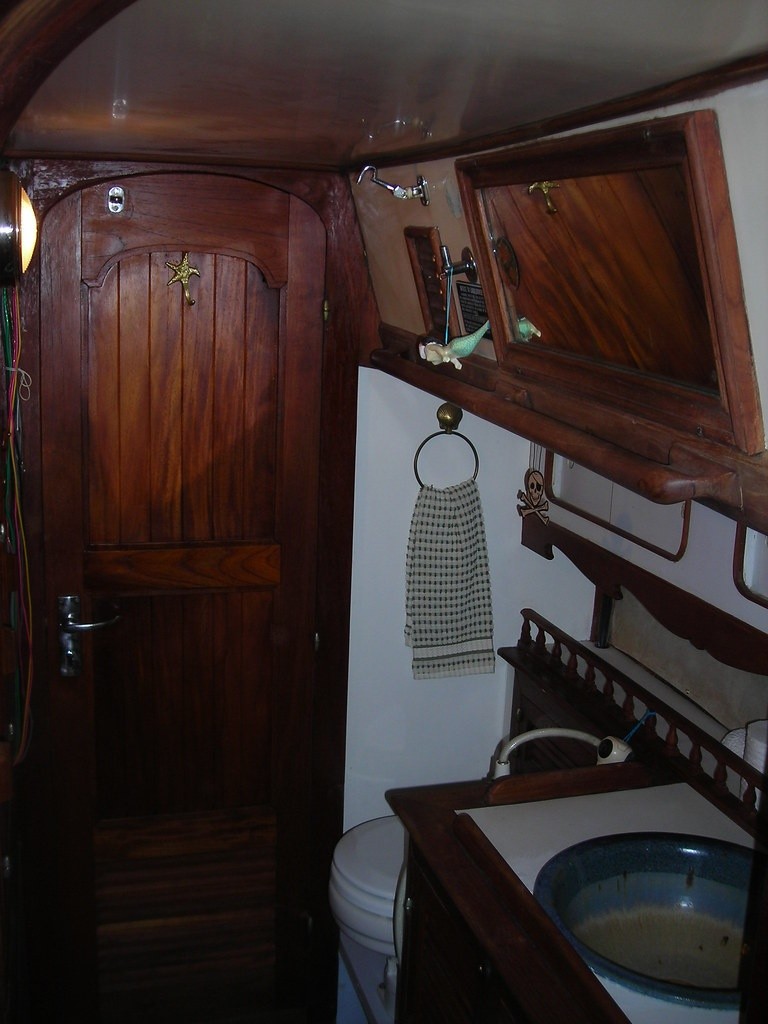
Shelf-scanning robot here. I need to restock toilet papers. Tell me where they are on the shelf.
[740,721,768,809]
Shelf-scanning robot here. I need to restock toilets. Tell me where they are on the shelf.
[331,815,404,959]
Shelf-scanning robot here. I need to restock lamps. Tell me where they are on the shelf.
[0,170,38,288]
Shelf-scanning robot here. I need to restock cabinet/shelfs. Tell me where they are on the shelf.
[394,833,516,1024]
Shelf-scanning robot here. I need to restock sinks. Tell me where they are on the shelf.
[533,831,756,1011]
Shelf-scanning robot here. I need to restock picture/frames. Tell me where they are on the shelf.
[404,224,462,340]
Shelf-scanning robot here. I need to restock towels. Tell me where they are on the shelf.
[723,728,746,758]
[404,478,496,683]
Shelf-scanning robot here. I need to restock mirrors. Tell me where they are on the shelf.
[452,106,762,455]
